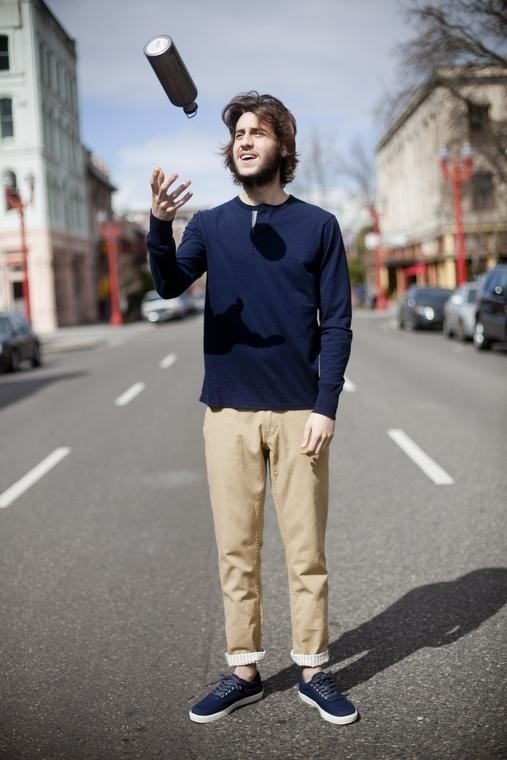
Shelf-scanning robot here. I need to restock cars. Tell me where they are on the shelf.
[398,264,507,351]
[0,312,40,372]
[140,290,205,322]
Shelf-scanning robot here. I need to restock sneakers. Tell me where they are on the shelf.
[297,668,359,725]
[189,669,264,724]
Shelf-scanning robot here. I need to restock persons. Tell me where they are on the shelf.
[145,86,363,728]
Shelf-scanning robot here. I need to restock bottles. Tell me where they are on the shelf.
[143,35,198,119]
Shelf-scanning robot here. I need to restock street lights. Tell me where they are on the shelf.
[93,205,128,324]
[438,138,473,286]
[3,169,36,330]
